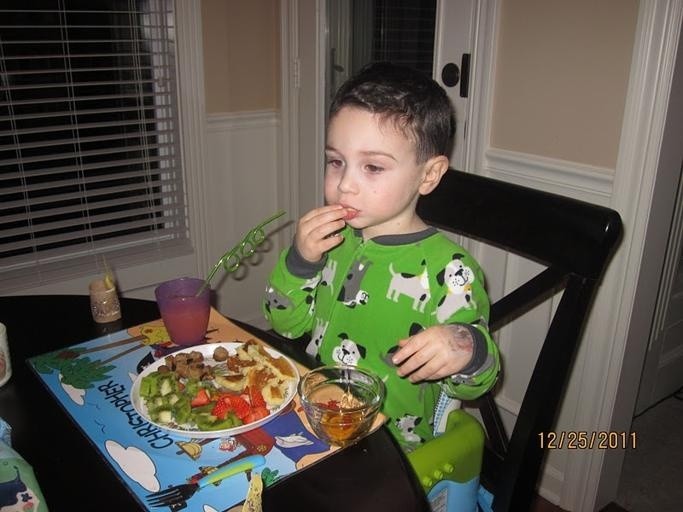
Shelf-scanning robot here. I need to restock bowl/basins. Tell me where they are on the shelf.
[296,366,385,446]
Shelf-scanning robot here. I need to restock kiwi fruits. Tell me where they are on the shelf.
[140,374,242,430]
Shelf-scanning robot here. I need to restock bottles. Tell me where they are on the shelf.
[88,279,122,324]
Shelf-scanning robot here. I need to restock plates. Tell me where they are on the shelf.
[127,340,301,438]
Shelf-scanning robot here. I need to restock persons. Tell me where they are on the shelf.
[262,55,507,453]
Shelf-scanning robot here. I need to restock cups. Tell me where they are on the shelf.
[155,276,213,346]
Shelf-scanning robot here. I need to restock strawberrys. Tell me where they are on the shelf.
[191,386,269,424]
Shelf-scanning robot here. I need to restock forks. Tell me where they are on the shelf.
[146,453,265,508]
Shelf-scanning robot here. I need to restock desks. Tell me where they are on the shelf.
[0,296,430,512]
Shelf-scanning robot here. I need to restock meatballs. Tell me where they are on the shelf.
[213,346,228,362]
[157,351,205,379]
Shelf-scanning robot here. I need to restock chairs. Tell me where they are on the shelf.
[266,162,624,510]
